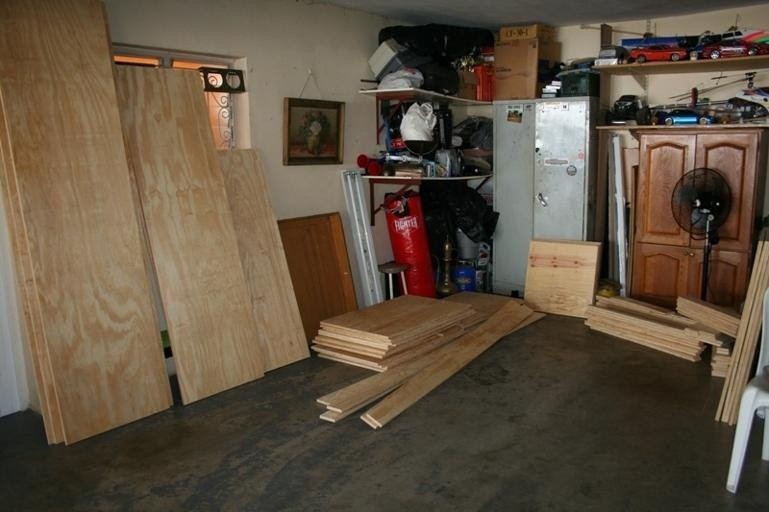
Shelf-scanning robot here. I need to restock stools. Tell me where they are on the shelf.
[377,262,409,300]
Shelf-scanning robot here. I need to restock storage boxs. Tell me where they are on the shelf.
[493,24,556,99]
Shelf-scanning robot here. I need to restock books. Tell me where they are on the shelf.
[541,93,557,98]
[541,87,558,94]
[551,79,561,86]
[545,84,561,89]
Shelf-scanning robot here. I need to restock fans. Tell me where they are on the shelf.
[671,167,732,302]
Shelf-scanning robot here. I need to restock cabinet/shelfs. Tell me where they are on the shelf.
[628,129,765,316]
[358,88,493,226]
[491,97,598,299]
[591,55,768,129]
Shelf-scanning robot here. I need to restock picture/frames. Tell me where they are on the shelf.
[282,95,345,166]
